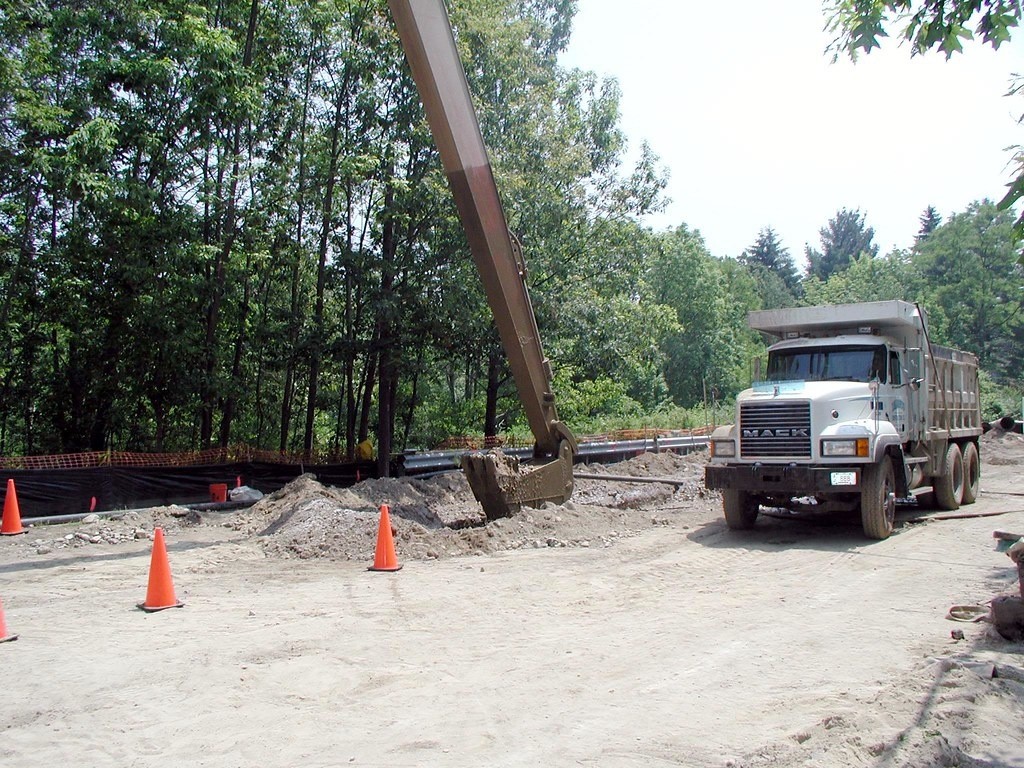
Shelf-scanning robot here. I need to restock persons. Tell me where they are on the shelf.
[354,435,373,461]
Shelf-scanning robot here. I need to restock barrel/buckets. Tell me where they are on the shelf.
[210,484,228,502]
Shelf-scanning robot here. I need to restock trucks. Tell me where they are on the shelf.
[706,304,979,541]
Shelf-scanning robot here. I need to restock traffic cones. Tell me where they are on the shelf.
[2,477,27,538]
[369,509,399,572]
[137,527,180,616]
